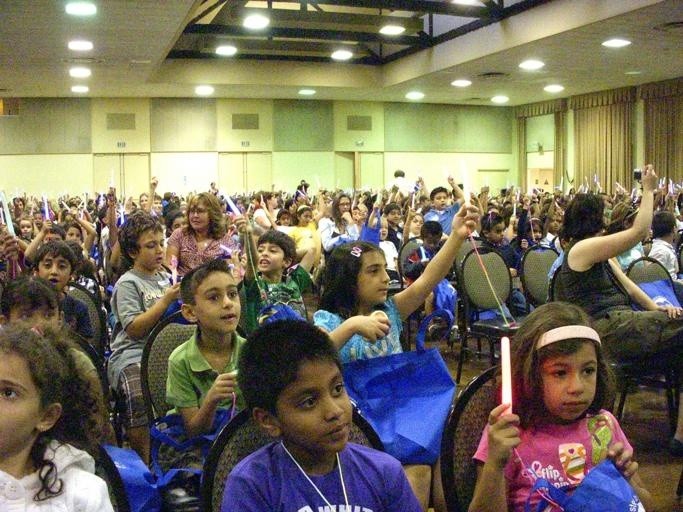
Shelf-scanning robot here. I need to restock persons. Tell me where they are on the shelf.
[253,180,345,198]
[165,193,247,287]
[166,182,252,208]
[423,175,466,236]
[234,217,317,335]
[158,259,252,477]
[110,209,185,464]
[646,210,680,282]
[314,198,483,512]
[480,212,536,320]
[655,173,683,218]
[468,301,652,510]
[403,208,448,243]
[33,240,93,345]
[404,221,458,325]
[345,169,431,207]
[271,209,292,227]
[552,164,683,458]
[220,316,424,511]
[0,177,165,258]
[0,320,120,512]
[319,194,360,263]
[608,202,646,278]
[285,200,299,226]
[382,202,406,250]
[467,175,570,212]
[167,208,186,239]
[367,202,402,281]
[580,174,640,230]
[514,196,545,276]
[1,276,116,462]
[260,193,322,266]
[252,192,281,228]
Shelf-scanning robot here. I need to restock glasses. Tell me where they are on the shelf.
[185,206,213,216]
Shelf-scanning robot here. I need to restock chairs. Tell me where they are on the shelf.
[627,257,682,316]
[545,265,682,441]
[396,236,465,350]
[454,235,486,328]
[456,246,521,383]
[67,333,103,392]
[65,436,131,511]
[519,243,563,313]
[440,360,620,512]
[198,399,384,512]
[69,284,105,349]
[143,307,249,463]
